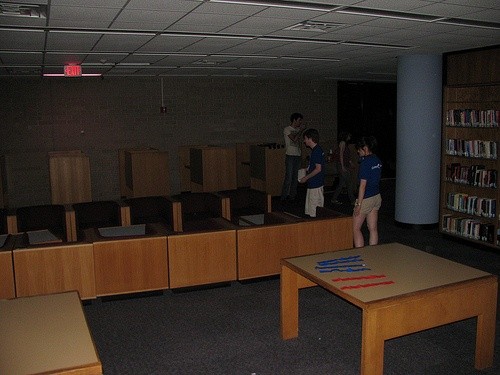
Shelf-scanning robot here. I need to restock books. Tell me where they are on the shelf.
[442,191,500,246]
[446,163,496,188]
[446,108,499,127]
[447,139,497,160]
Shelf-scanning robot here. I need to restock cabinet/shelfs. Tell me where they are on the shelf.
[438,44,500,255]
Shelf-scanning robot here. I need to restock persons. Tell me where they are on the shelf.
[280,112,306,200]
[330,130,352,203]
[303,129,325,217]
[351,135,382,248]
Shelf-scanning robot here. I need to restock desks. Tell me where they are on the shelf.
[0,289,103,375]
[279,242,500,375]
[0,194,354,306]
